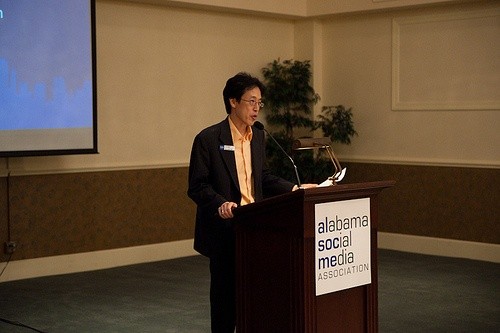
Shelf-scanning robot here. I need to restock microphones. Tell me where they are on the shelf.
[254,121,301,187]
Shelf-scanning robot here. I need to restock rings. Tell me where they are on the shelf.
[221,210,224,212]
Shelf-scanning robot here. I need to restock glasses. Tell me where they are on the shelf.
[241,98,264,107]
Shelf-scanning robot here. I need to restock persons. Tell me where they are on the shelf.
[186,71,318,333]
